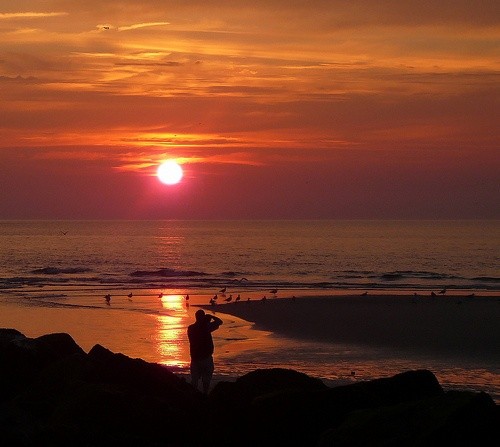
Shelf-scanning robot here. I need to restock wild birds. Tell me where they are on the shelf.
[431,291,436,296]
[218,288,226,294]
[209,298,216,303]
[240,277,249,283]
[269,288,278,294]
[361,291,368,296]
[248,298,250,301]
[159,293,163,298]
[213,294,217,300]
[223,295,232,302]
[235,295,241,302]
[440,287,447,293]
[128,292,133,297]
[293,296,296,298]
[186,294,189,300]
[232,278,238,282]
[261,296,266,301]
[104,294,111,299]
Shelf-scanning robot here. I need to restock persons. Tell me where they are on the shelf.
[187,309,223,396]
